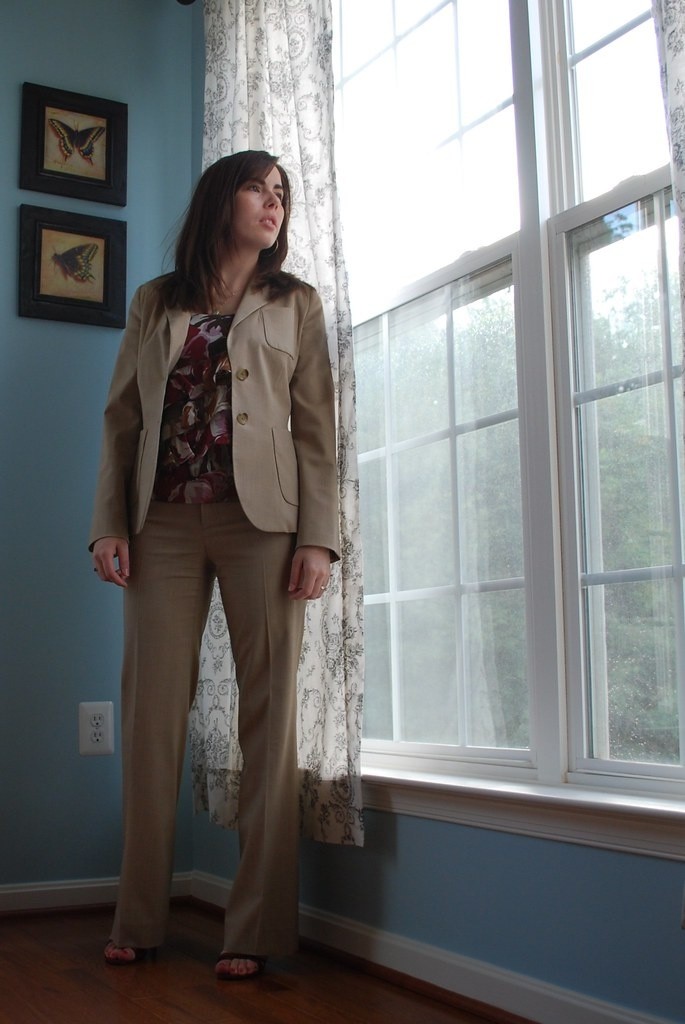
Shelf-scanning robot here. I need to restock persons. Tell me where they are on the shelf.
[86,151,342,980]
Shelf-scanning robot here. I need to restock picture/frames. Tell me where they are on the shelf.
[14,199,133,330]
[16,80,132,209]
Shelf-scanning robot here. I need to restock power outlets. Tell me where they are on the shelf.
[77,701,116,757]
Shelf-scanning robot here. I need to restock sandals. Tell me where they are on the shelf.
[214,952,267,980]
[104,938,150,965]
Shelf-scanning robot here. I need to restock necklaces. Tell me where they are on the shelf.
[210,286,243,315]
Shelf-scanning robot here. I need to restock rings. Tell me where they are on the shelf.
[93,568,98,572]
[320,585,327,590]
